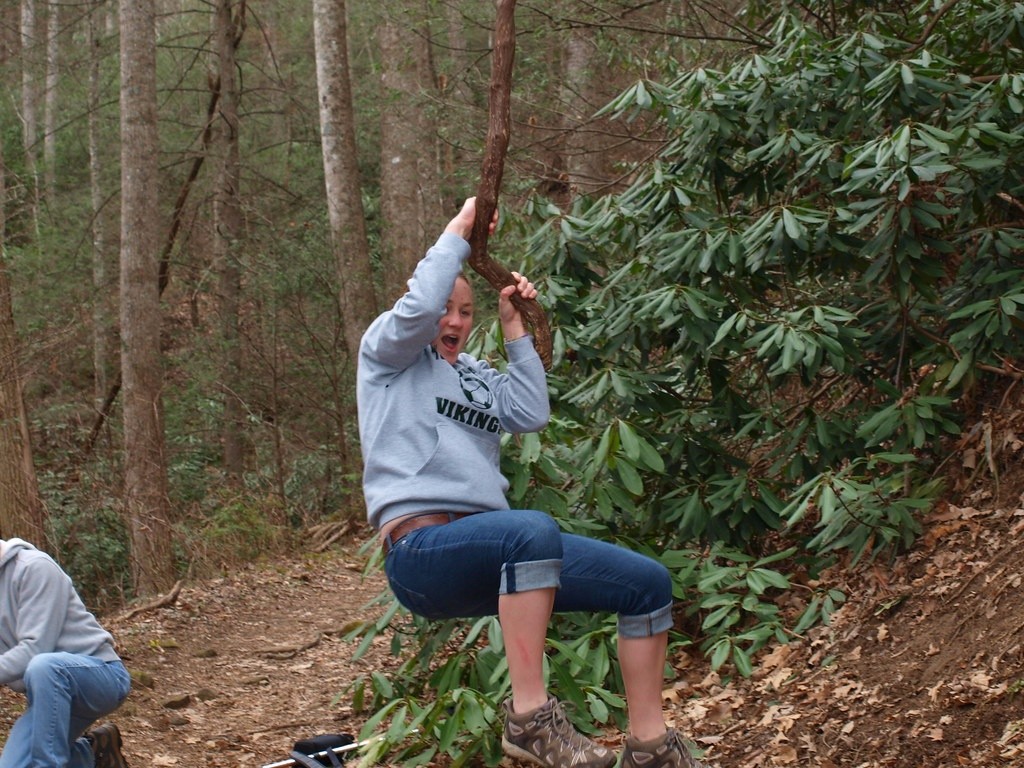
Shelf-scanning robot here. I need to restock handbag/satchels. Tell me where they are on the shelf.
[289,733,359,768]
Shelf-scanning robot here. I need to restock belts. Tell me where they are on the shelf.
[381,512,465,558]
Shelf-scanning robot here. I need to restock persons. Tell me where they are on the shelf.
[357,197,700,768]
[0,537,129,768]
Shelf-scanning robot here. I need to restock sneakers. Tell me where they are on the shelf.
[621,723,709,768]
[502,692,617,768]
[82,722,130,768]
